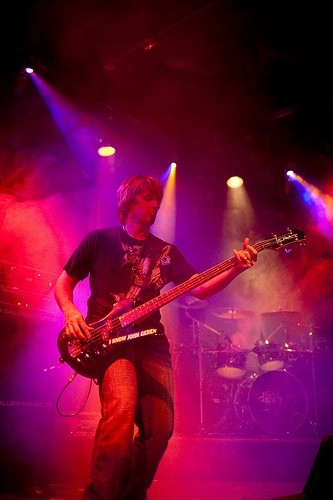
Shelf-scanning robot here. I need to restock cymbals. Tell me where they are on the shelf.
[211,308,256,320]
[174,300,209,308]
[262,310,299,317]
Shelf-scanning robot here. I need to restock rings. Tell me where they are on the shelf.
[243,259,248,261]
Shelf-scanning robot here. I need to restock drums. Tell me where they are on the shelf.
[234,370,308,434]
[258,351,283,371]
[213,350,247,378]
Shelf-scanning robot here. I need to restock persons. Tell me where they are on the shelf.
[54,176,257,500]
[230,317,261,350]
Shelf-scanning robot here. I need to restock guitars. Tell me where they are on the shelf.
[57,228,307,378]
